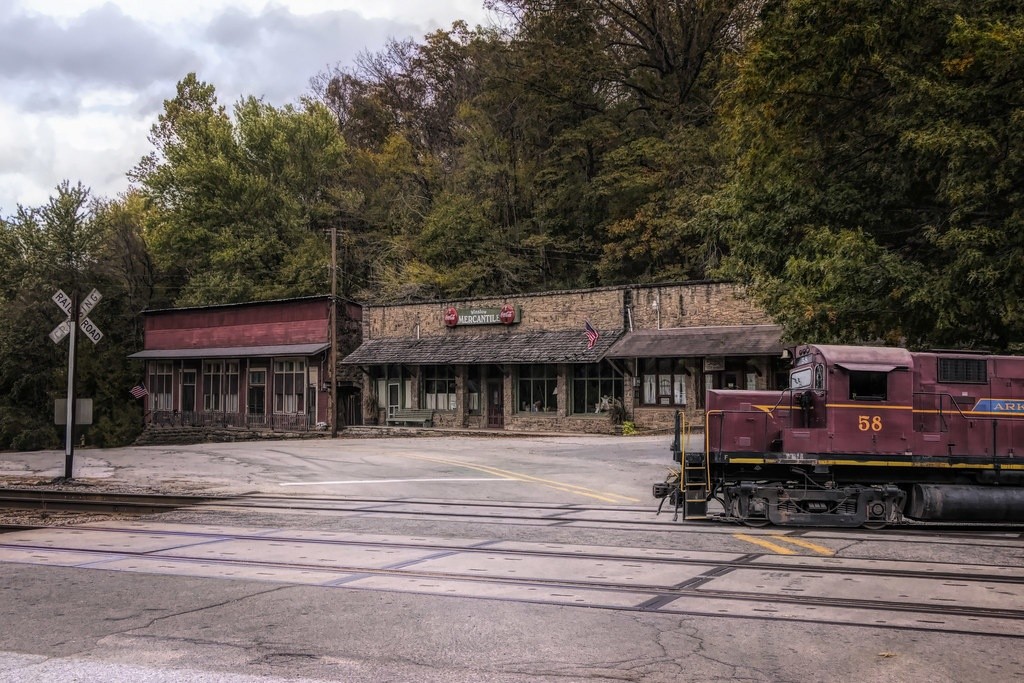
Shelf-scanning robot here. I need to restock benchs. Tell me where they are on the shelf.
[386,409,435,428]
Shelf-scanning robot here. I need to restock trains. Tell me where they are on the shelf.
[652,344,1024,528]
